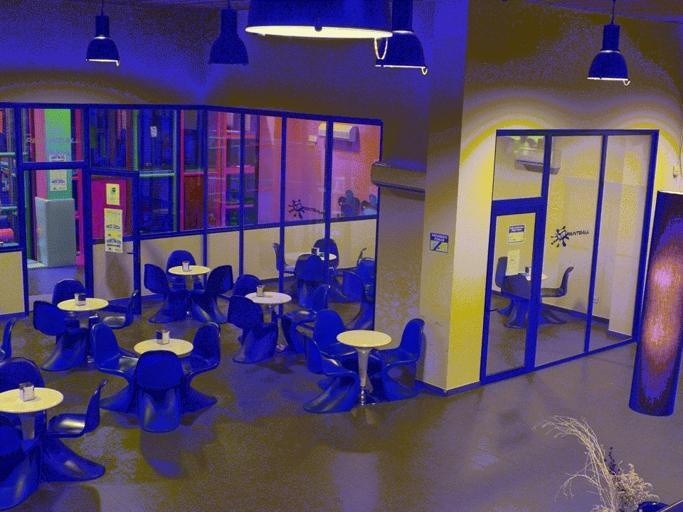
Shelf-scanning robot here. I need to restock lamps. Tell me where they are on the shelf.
[83,0,120,66]
[375,0,426,69]
[208,0,249,66]
[586,0,629,81]
[244,0,393,40]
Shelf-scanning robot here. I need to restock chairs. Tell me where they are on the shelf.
[495,256,574,329]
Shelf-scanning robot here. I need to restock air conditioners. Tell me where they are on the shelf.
[371,161,426,195]
[512,146,561,169]
[318,122,358,142]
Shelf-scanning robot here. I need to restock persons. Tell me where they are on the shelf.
[337,190,377,217]
